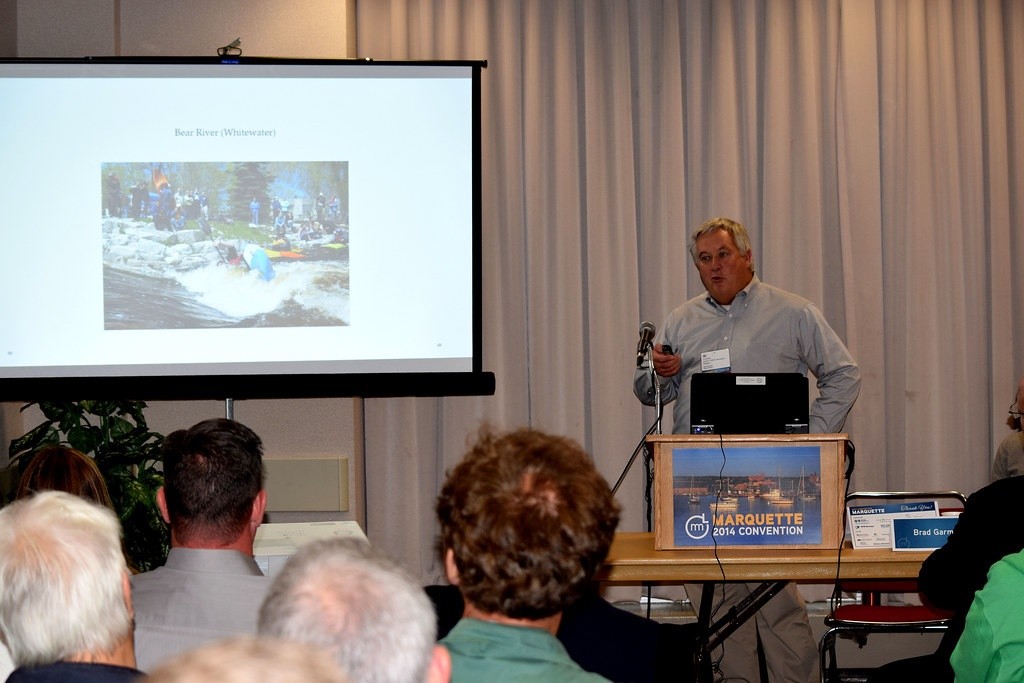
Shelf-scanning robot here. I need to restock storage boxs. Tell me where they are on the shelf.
[644,434,848,548]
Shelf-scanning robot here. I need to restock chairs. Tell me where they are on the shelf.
[816,487,965,683]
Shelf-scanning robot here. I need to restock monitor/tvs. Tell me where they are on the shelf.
[689,372,808,435]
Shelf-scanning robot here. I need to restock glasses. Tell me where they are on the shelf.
[1009,402,1023,419]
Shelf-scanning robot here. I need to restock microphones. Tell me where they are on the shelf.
[637,321,655,365]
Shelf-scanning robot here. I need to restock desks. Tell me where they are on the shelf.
[603,530,937,683]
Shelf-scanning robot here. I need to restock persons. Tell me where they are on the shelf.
[633,217,861,682]
[106,171,341,270]
[0,416,708,680]
[866,369,1024,683]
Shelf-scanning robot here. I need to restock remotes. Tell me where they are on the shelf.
[662,345,674,355]
